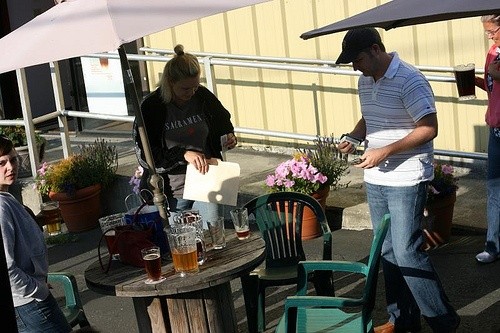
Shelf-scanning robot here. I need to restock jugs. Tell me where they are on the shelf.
[172,209,207,266]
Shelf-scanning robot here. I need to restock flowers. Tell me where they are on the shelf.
[426,157,460,203]
[260,133,352,195]
[27,135,119,195]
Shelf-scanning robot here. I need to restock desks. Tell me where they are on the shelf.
[84,229,266,333]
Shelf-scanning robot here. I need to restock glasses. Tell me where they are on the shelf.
[485,26,500,38]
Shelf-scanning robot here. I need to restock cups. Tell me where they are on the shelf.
[206,217,226,250]
[163,223,206,275]
[453,63,477,101]
[41,200,61,236]
[230,208,250,240]
[99,213,125,261]
[140,246,161,282]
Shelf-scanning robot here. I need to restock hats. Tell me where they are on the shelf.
[335,27,381,65]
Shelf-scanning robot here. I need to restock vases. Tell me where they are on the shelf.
[424,186,456,246]
[275,187,329,240]
[48,183,103,232]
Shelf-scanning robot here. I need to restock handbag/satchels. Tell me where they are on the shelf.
[124,189,169,253]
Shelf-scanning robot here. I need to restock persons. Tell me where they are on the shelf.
[474,15,500,262]
[132,44,237,212]
[336,28,460,333]
[0,133,74,333]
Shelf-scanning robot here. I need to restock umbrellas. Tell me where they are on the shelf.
[300,0,500,41]
[0,0,277,229]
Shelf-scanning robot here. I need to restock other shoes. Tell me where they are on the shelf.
[429,314,461,333]
[475,250,497,262]
[372,318,422,333]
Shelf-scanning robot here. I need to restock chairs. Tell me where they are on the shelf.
[47,272,91,329]
[274,213,392,333]
[240,192,336,333]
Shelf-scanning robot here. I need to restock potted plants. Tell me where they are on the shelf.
[0,118,46,177]
